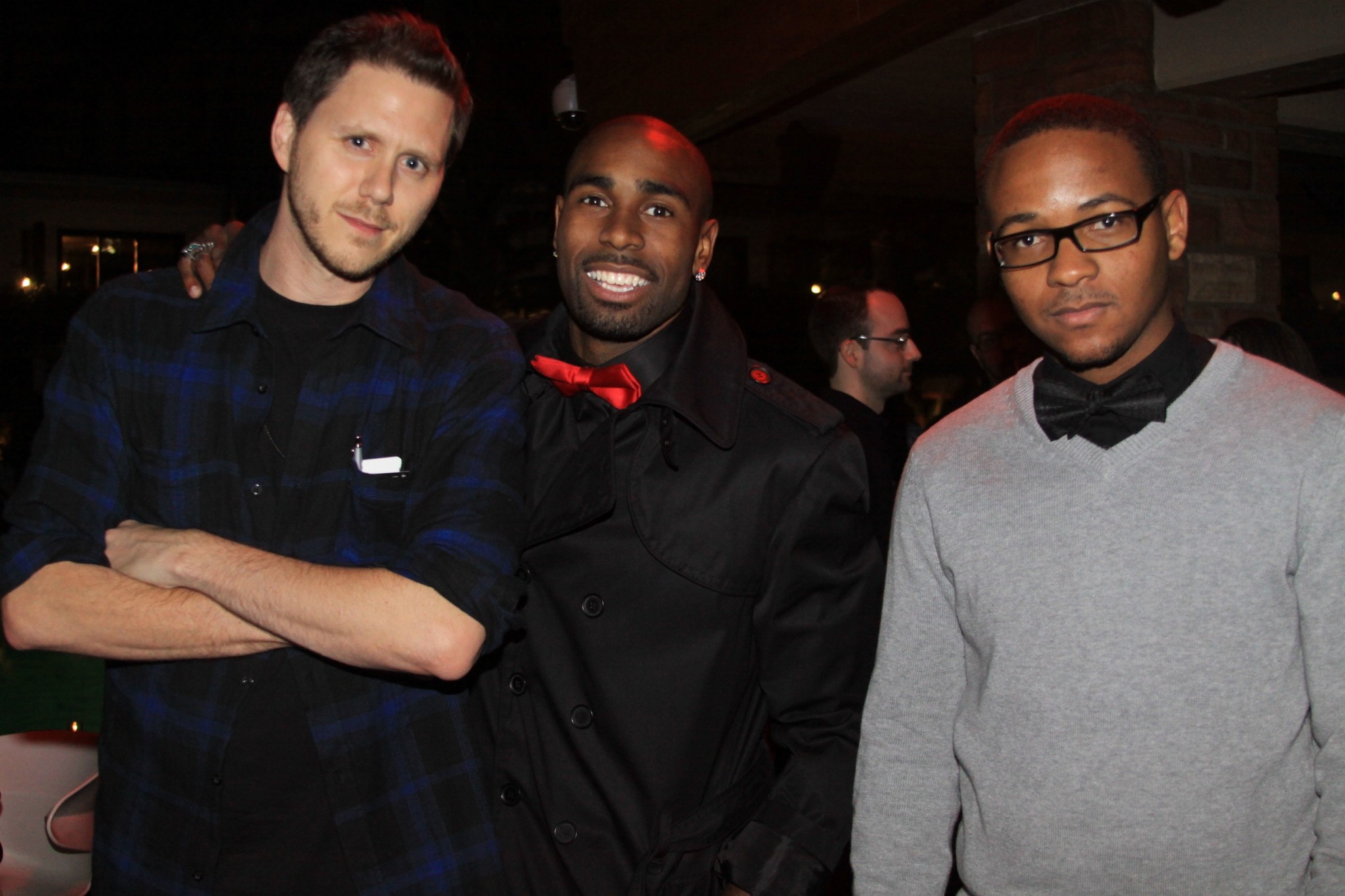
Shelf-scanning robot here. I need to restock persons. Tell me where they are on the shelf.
[0,11,522,896]
[822,279,1303,562]
[850,92,1345,896]
[180,114,879,895]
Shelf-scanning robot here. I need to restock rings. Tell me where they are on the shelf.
[183,238,214,262]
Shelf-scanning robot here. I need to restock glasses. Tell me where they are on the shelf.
[851,334,909,351]
[991,193,1163,269]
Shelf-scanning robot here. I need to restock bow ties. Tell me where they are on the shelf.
[1032,360,1169,442]
[531,355,641,410]
